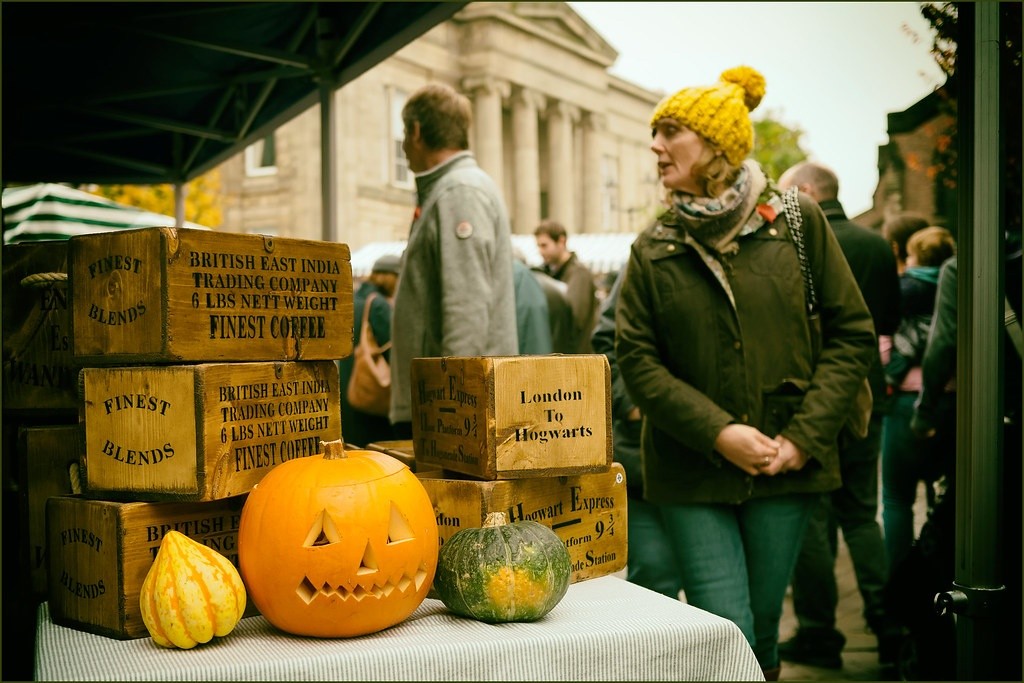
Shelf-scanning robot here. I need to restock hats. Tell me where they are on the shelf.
[651,65,765,166]
[373,255,401,275]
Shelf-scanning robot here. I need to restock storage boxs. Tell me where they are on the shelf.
[411,461,629,600]
[4,238,81,622]
[79,365,344,505]
[45,494,264,642]
[411,354,613,480]
[67,227,356,361]
[366,439,418,468]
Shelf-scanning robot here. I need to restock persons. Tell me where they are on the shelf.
[590,263,687,602]
[387,83,523,432]
[344,199,1006,560]
[613,63,875,683]
[766,161,891,668]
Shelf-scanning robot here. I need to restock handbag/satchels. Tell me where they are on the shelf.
[347,321,391,418]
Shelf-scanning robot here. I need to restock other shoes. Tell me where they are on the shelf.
[777,626,847,669]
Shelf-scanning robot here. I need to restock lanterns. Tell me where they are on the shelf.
[238,438,441,643]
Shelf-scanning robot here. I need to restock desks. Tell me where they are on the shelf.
[34,573,770,683]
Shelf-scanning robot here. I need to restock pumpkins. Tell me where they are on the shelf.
[238,439,442,641]
[139,528,248,650]
[427,509,573,625]
